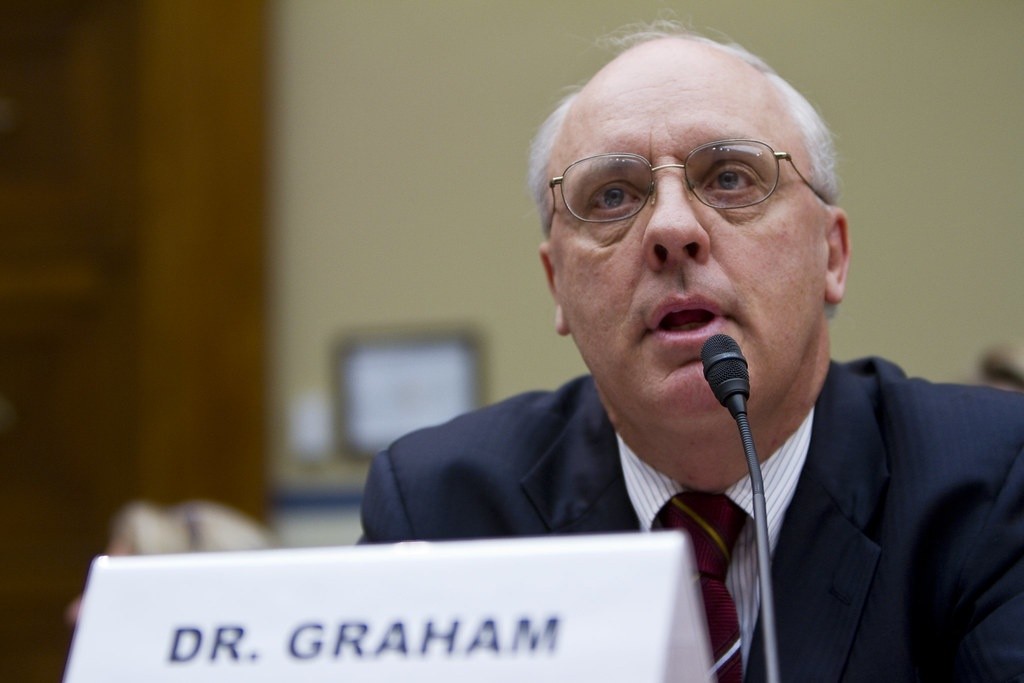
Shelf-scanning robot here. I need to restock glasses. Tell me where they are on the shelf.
[548,139,831,238]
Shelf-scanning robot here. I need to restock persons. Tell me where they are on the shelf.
[357,20,1024,683]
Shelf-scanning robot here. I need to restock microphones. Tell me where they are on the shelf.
[700,334,780,683]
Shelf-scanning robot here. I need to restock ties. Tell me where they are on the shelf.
[655,489,750,683]
